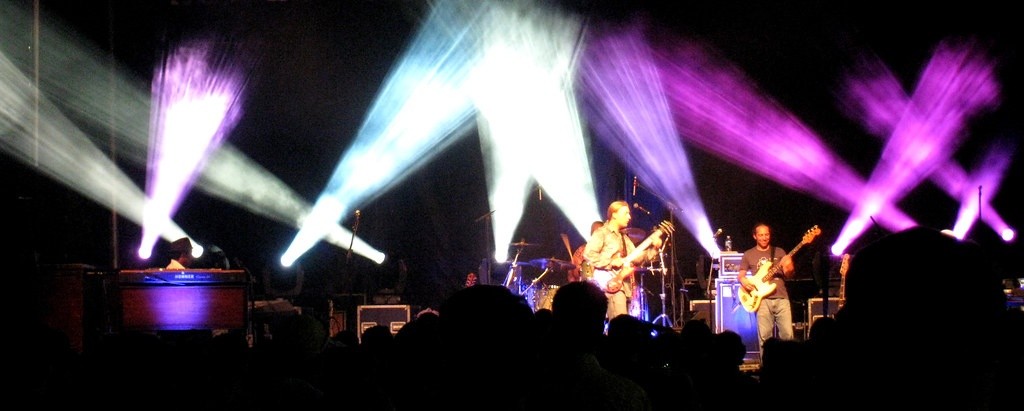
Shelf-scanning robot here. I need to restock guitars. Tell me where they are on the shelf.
[737,223,823,313]
[593,221,675,295]
[837,252,851,310]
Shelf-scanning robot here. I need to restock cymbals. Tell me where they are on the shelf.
[527,257,577,272]
[619,226,647,245]
[508,241,535,247]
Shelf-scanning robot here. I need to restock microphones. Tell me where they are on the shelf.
[355,209,364,231]
[633,203,650,216]
[712,229,723,239]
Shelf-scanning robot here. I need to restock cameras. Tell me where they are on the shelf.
[602,318,608,335]
[651,329,658,337]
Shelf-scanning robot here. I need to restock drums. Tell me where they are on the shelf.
[534,284,561,310]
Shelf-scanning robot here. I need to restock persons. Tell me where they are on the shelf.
[737,224,795,368]
[0,227,1024,411]
[568,200,662,324]
